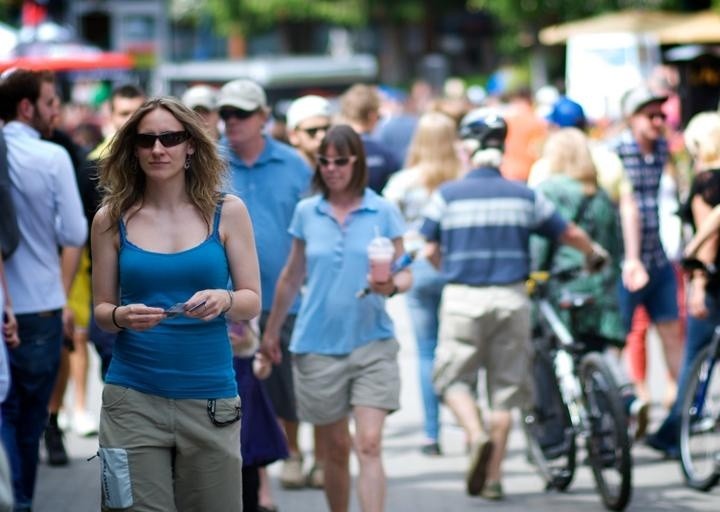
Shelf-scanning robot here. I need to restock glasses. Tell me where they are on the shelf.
[133,128,193,148]
[313,153,358,168]
[218,109,253,119]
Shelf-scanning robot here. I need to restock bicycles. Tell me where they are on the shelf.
[674,253,720,495]
[516,254,638,512]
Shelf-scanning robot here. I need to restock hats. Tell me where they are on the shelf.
[621,86,668,117]
[286,95,331,131]
[544,99,584,127]
[217,79,266,112]
[180,84,216,113]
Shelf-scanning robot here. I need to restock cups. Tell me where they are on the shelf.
[368,237,395,285]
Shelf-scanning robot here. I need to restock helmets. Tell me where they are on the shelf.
[457,110,507,150]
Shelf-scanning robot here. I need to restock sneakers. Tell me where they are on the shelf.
[308,465,325,488]
[44,428,68,465]
[467,433,494,496]
[623,389,647,441]
[278,456,305,488]
[69,408,100,439]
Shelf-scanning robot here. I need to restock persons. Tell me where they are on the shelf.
[1,70,720,510]
